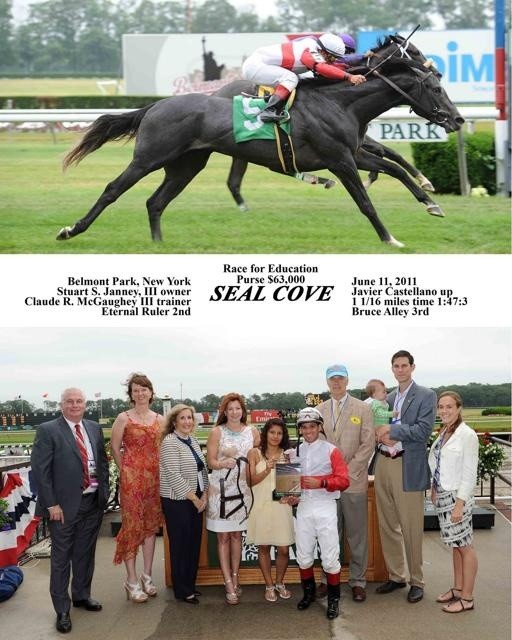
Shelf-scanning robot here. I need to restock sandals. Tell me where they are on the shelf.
[273,582,293,599]
[224,579,238,605]
[230,571,244,597]
[433,587,462,603]
[264,584,278,603]
[441,597,475,613]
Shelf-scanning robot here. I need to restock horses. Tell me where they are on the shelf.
[209,30,442,213]
[55,57,465,250]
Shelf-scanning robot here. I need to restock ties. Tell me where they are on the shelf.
[334,401,340,417]
[72,424,91,493]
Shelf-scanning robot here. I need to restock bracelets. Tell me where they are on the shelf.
[321,480,327,489]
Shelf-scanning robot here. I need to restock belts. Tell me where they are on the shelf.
[376,448,403,459]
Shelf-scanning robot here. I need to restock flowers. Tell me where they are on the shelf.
[104,439,120,491]
[426,428,507,485]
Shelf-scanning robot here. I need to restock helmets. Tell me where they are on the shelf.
[295,406,324,426]
[316,32,356,59]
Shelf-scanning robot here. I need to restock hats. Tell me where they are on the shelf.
[325,363,349,380]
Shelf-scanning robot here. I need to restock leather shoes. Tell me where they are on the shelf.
[72,595,104,612]
[352,586,368,603]
[406,585,424,604]
[375,579,407,595]
[314,581,328,598]
[54,609,73,633]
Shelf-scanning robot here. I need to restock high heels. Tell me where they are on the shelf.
[173,589,203,605]
[136,572,159,597]
[122,580,149,603]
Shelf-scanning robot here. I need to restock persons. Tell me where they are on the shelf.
[245,419,294,602]
[110,370,166,604]
[29,387,109,634]
[240,33,367,126]
[312,363,375,602]
[290,35,375,66]
[283,407,350,620]
[363,378,406,459]
[204,392,261,606]
[155,402,212,605]
[428,390,479,615]
[375,349,438,603]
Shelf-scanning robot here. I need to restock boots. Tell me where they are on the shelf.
[325,583,341,619]
[293,575,317,610]
[260,94,291,124]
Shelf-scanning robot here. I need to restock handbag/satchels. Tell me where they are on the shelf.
[0,565,24,603]
